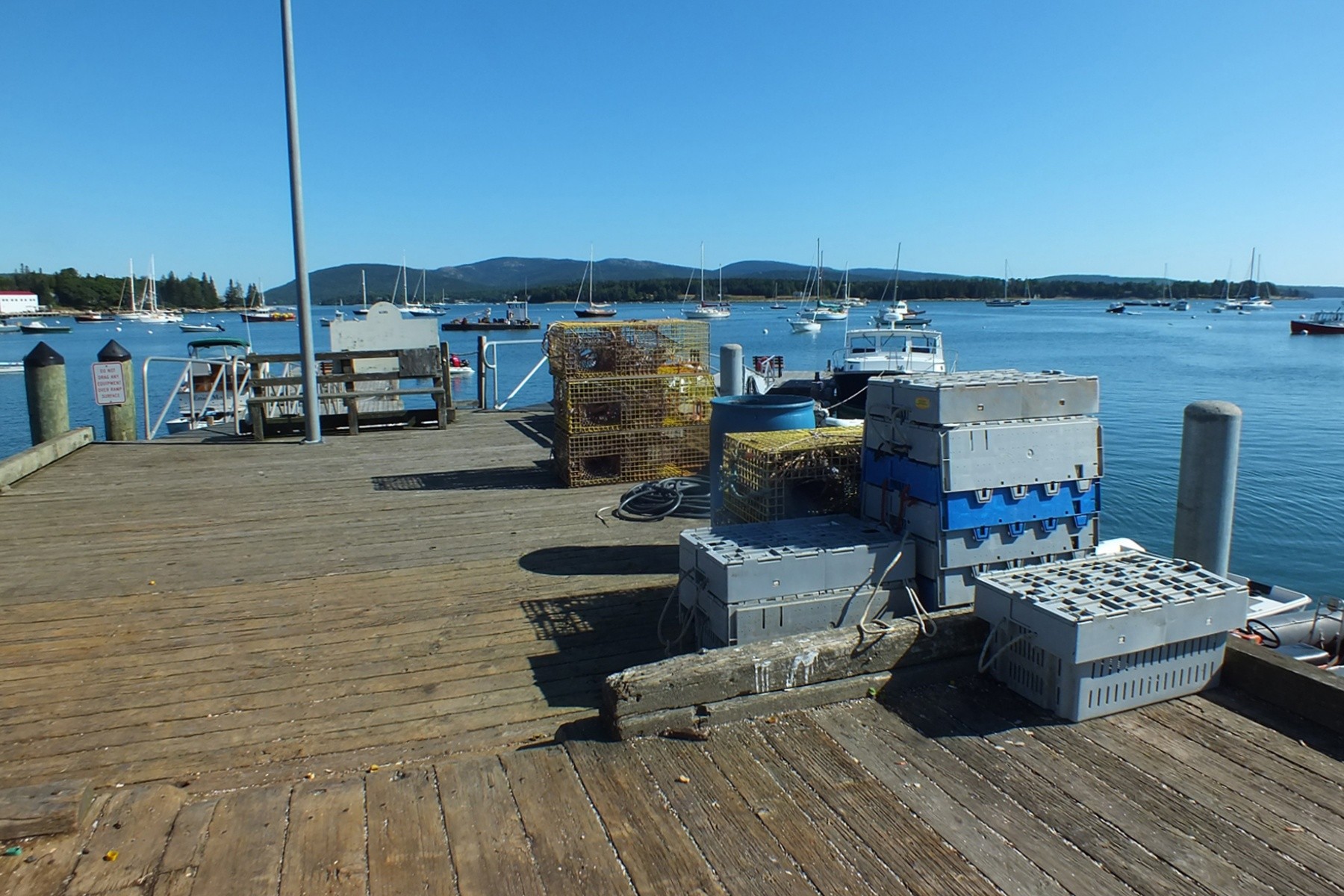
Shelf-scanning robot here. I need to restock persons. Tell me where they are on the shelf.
[449,353,461,367]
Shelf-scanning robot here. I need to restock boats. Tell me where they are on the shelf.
[0,321,73,334]
[166,285,413,433]
[238,277,299,322]
[1171,299,1192,312]
[73,310,115,323]
[1104,298,1149,314]
[1290,297,1344,336]
[441,276,540,331]
[319,308,346,327]
[827,313,946,422]
[179,323,222,333]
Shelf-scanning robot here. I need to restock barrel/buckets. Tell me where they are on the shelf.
[708,394,815,528]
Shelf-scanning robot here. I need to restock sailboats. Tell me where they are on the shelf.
[350,247,451,317]
[113,250,186,324]
[984,260,1025,307]
[788,237,870,332]
[1152,262,1178,307]
[769,281,787,310]
[875,241,932,327]
[572,244,619,318]
[1210,247,1274,313]
[679,239,733,318]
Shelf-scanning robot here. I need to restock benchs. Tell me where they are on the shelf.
[244,345,446,440]
